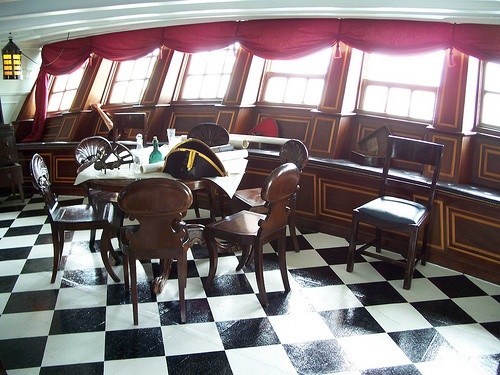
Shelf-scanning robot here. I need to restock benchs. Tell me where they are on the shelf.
[16,141,500,287]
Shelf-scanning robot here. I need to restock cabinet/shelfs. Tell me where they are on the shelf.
[0,99,25,203]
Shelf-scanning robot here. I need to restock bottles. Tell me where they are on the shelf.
[149,136,163,164]
[133,133,145,174]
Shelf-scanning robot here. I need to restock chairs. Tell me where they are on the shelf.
[76,135,129,250]
[187,123,229,218]
[202,162,299,306]
[31,153,122,283]
[347,135,445,290]
[118,176,205,325]
[222,140,309,266]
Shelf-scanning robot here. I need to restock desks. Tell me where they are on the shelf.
[75,148,241,283]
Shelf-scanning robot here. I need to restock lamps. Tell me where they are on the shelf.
[2,30,69,80]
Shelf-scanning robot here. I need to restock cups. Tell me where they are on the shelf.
[167,129,187,150]
